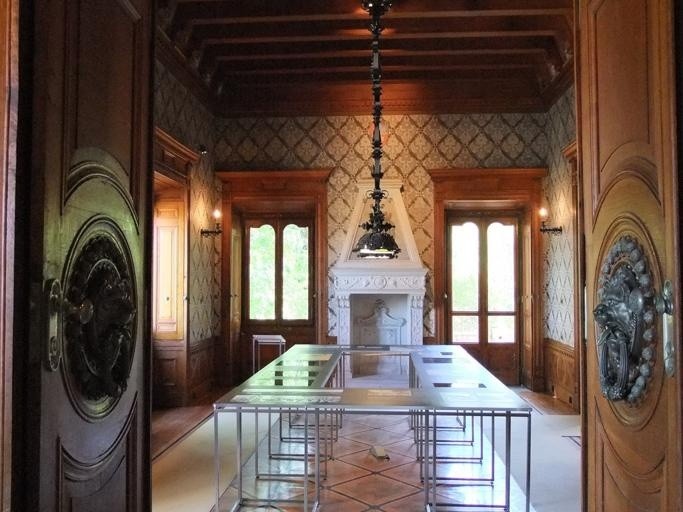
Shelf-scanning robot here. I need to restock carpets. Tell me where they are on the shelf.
[152,407,282,512]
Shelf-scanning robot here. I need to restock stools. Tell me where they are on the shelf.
[252,334,287,375]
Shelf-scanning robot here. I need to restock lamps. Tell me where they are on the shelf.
[538,207,562,233]
[352,0,400,260]
[201,209,223,234]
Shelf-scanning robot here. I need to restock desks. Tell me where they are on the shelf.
[213,342,532,512]
